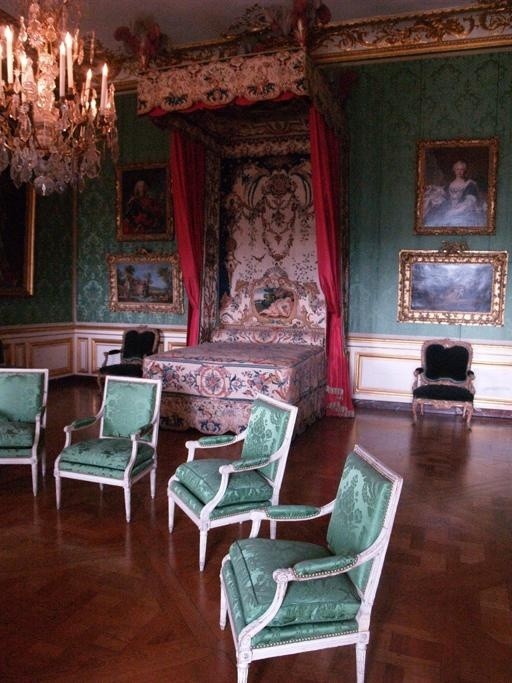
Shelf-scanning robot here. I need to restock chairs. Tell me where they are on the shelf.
[0,368,49,496]
[98,325,159,394]
[412,338,476,429]
[166,394,299,573]
[53,375,162,523]
[220,443,404,683]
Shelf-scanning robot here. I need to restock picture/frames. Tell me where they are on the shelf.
[114,164,174,242]
[396,238,508,328]
[415,137,496,236]
[0,178,35,297]
[107,249,184,314]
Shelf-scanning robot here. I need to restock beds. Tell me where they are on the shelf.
[114,0,361,439]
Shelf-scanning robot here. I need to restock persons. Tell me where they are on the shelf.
[125,180,159,229]
[425,160,482,228]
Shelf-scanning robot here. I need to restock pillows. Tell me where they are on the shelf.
[211,328,327,346]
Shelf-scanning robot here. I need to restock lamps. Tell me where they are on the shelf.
[0,1,120,195]
